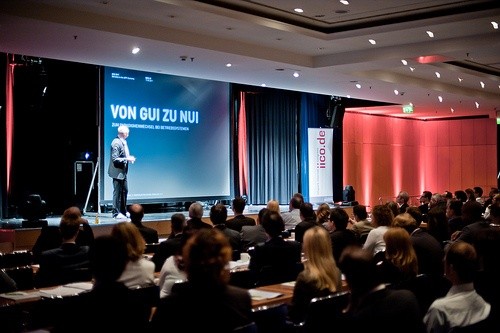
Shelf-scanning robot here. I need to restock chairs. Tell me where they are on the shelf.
[0,232,442,333]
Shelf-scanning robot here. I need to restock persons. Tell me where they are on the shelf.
[108,126,135,218]
[31,186,500,333]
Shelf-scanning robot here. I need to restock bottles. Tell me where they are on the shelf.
[95,213,99,224]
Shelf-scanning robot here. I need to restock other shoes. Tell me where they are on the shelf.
[113,212,130,219]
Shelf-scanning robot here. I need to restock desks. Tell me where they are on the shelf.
[243,281,302,307]
[0,272,168,312]
